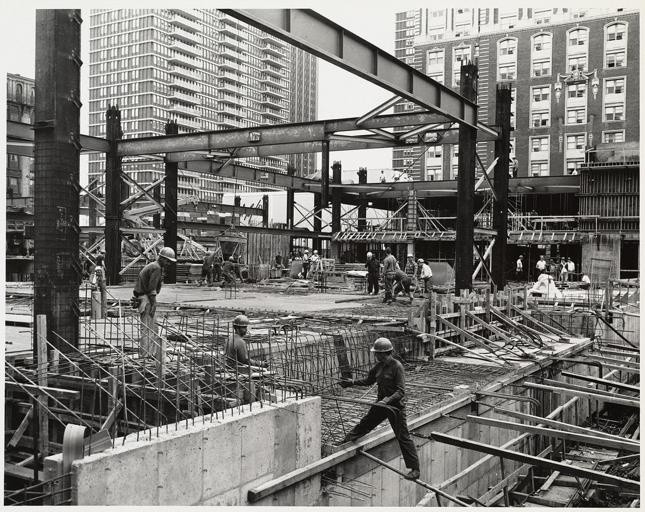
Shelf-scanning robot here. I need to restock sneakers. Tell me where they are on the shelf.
[332,441,346,446]
[404,470,420,479]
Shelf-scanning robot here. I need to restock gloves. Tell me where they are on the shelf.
[378,397,391,405]
[337,377,354,389]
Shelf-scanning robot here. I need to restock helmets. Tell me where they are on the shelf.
[158,247,177,262]
[233,315,250,327]
[539,255,570,262]
[367,252,372,258]
[229,256,234,261]
[304,250,318,254]
[519,255,523,258]
[369,338,393,352]
[407,253,424,263]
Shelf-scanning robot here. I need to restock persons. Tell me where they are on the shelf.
[223,314,268,369]
[331,337,420,480]
[134,247,177,360]
